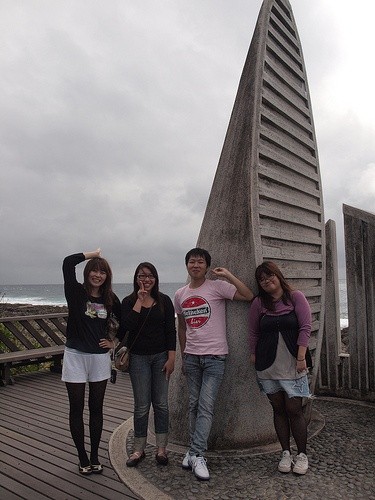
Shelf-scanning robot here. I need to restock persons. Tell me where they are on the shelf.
[248,261,312,475]
[174,247,254,481]
[62,247,122,474]
[122,262,177,467]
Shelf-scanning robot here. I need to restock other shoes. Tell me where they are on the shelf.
[155,447,168,465]
[126,449,146,467]
[89,459,103,473]
[79,460,92,473]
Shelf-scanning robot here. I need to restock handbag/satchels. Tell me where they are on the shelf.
[114,342,130,373]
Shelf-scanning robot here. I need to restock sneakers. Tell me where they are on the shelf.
[188,455,210,479]
[292,453,308,475]
[182,448,191,469]
[278,450,293,472]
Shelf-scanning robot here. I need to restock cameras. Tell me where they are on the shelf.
[110,369,117,384]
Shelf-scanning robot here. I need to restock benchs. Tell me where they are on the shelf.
[0,345,65,386]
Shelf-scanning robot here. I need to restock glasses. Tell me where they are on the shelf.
[137,274,155,280]
[259,273,275,284]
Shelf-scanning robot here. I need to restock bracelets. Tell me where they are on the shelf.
[297,358,305,361]
